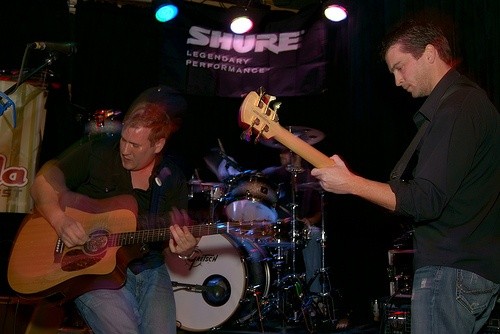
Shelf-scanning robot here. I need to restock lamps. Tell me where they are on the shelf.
[63,0,81,17]
[323,0,351,24]
[224,6,257,34]
[153,0,180,25]
[113,0,129,8]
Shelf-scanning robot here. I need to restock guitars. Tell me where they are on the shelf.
[7,209,312,300]
[240,85,338,169]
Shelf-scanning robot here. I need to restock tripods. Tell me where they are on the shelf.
[251,148,340,334]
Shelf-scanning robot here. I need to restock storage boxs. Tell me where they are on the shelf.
[386,249,417,299]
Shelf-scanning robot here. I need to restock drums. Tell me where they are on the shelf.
[192,182,225,224]
[162,231,270,330]
[225,174,278,222]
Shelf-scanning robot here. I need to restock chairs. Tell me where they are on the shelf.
[24,299,92,334]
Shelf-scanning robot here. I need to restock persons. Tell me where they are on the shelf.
[311,23,500,334]
[30,98,201,334]
[260,150,322,235]
[84,109,121,132]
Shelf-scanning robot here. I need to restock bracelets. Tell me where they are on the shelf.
[177,251,195,260]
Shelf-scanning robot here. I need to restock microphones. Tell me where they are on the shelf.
[220,151,243,172]
[32,41,77,56]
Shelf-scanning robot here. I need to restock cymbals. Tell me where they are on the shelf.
[297,180,323,192]
[260,241,296,246]
[257,126,326,148]
